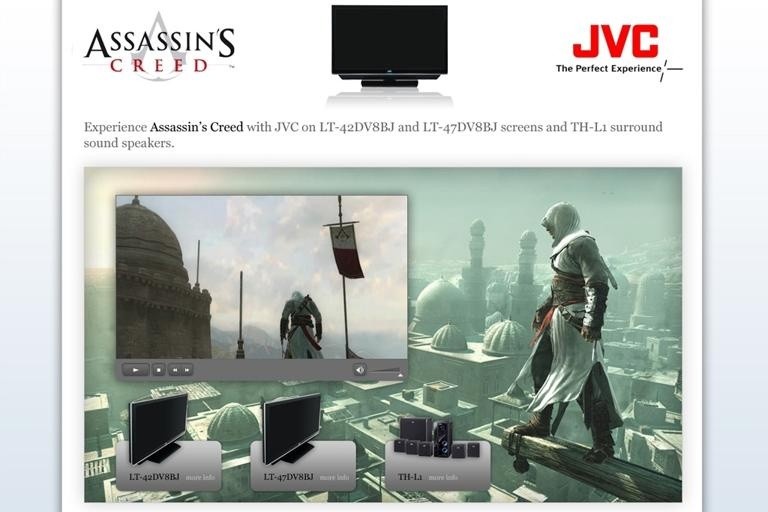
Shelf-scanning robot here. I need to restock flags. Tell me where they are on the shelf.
[330,226,364,280]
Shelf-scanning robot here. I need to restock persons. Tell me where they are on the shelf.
[279,291,324,359]
[510,202,625,465]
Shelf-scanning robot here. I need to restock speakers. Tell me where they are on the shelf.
[394,417,480,459]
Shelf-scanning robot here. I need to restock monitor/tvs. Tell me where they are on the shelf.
[128,392,188,466]
[332,5,452,100]
[263,393,321,467]
